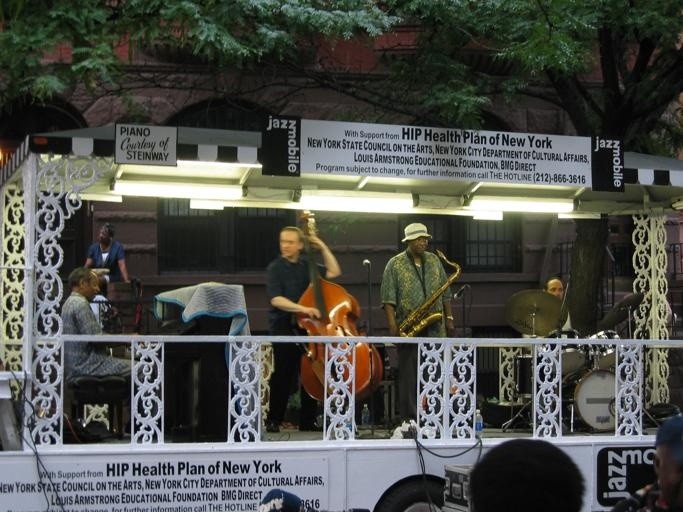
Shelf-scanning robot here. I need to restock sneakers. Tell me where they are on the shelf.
[300,422,318,431]
[265,422,278,432]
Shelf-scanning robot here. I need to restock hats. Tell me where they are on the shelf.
[401,223,432,242]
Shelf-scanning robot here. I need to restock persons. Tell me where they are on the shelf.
[466,439,585,511]
[83,221,131,283]
[380,222,457,426]
[262,226,342,432]
[523,276,572,352]
[91,274,111,327]
[610,415,683,512]
[61,267,154,433]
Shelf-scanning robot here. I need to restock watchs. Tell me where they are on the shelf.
[447,315,454,321]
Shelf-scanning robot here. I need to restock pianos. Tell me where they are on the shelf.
[126,282,248,442]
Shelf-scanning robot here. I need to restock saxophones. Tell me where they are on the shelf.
[397,249,462,337]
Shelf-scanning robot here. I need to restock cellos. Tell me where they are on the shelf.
[295,210,383,403]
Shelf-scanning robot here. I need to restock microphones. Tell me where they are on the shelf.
[454,285,465,301]
[362,259,371,266]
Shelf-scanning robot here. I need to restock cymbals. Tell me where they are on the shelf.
[502,290,569,336]
[601,293,645,329]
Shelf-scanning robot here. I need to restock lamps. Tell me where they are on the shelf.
[293,188,419,210]
[109,178,247,199]
[460,194,579,213]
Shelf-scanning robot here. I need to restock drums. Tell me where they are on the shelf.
[560,368,630,432]
[584,329,627,372]
[537,327,585,377]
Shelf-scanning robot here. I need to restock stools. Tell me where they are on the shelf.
[67,375,126,440]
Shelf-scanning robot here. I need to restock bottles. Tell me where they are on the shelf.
[476,409,484,438]
[360,403,370,425]
